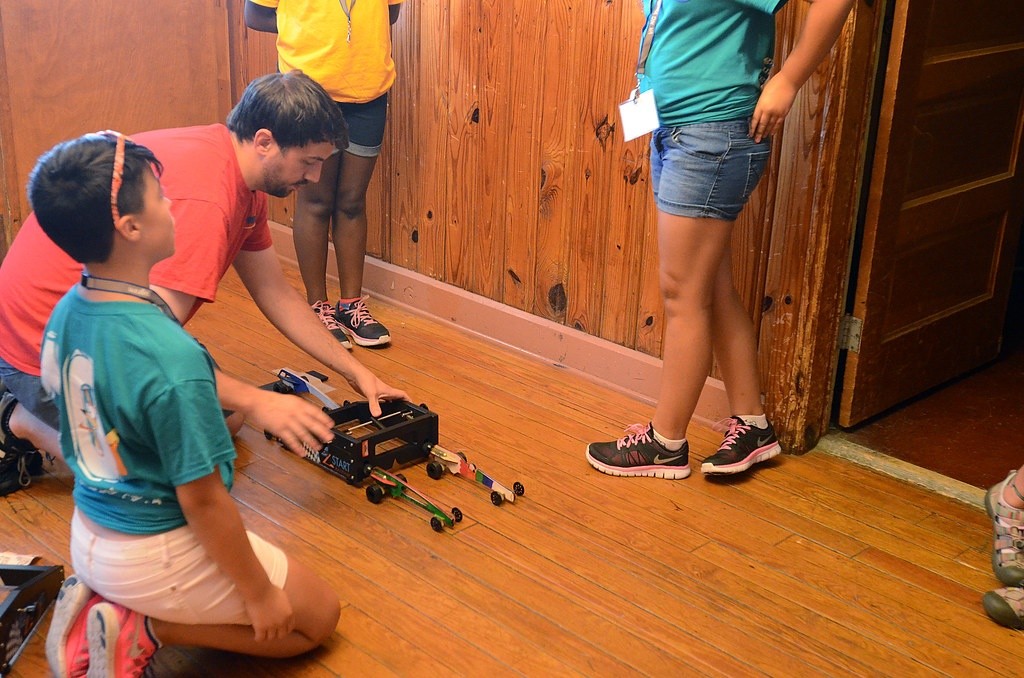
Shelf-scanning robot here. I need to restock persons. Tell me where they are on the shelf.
[983,465,1024,627]
[585,0,854,481]
[28,129,340,677]
[245,0,403,350]
[0,72,411,496]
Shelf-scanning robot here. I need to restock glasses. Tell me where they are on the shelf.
[92,130,133,232]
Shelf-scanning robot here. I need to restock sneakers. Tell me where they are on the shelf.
[983,467,1024,584]
[45,574,110,678]
[585,419,691,478]
[335,295,391,346]
[981,585,1024,631]
[699,414,781,476]
[0,388,41,496]
[85,601,160,678]
[311,300,353,349]
